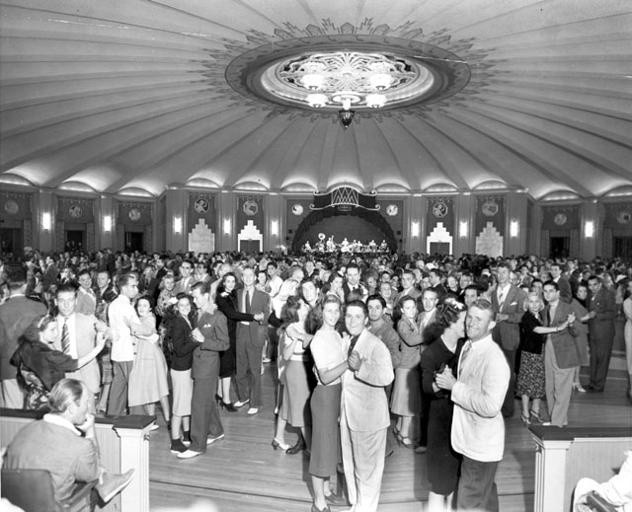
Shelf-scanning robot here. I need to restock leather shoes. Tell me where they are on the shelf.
[247,406,263,415]
[287,439,306,454]
[310,503,331,512]
[149,424,224,459]
[587,387,604,392]
[325,493,345,505]
[234,398,249,408]
[96,468,135,503]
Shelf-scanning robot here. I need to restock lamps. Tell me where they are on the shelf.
[289,49,404,111]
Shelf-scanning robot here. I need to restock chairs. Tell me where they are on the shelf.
[578,489,615,512]
[1,462,99,512]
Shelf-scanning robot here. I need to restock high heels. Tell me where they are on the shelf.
[530,410,544,423]
[96,403,107,414]
[520,412,531,425]
[215,394,222,403]
[574,382,586,393]
[220,400,237,412]
[272,438,291,450]
[397,433,417,449]
[393,427,399,438]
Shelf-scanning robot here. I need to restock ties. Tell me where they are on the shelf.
[499,289,503,305]
[61,318,72,356]
[245,290,251,314]
[348,337,359,356]
[459,344,471,374]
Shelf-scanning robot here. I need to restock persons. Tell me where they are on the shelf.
[269,246,629,511]
[2,378,136,512]
[1,244,273,459]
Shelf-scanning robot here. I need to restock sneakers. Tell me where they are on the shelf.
[416,446,426,454]
